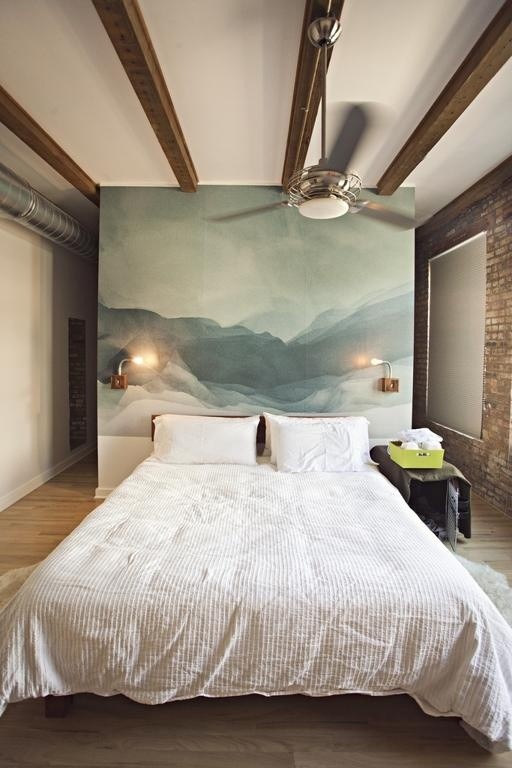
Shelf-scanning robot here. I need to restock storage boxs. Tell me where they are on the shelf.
[388,440,444,469]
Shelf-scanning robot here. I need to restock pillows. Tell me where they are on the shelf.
[154,413,369,473]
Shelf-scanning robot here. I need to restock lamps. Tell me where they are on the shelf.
[109,352,156,389]
[370,355,400,392]
[292,15,355,221]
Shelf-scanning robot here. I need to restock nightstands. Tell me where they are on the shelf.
[371,449,474,550]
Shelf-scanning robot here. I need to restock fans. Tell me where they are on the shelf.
[206,17,420,228]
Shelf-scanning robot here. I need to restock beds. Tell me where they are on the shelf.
[13,410,493,718]
